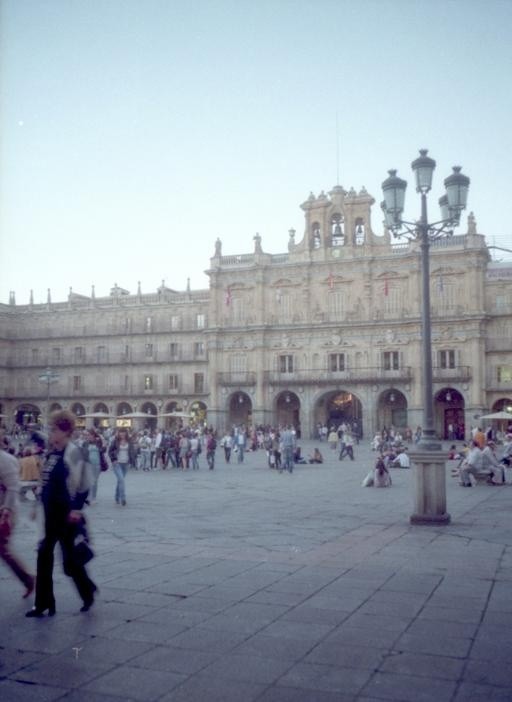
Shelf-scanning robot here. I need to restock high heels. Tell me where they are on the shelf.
[80,583,101,612]
[27,599,55,618]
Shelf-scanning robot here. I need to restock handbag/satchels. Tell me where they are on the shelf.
[361,471,375,488]
[66,523,94,567]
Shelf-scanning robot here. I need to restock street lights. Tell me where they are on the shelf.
[380,149,470,524]
[38,366,59,408]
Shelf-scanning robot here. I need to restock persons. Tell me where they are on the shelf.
[221,423,323,473]
[365,421,424,488]
[317,418,360,461]
[25,409,100,617]
[0,417,218,471]
[0,447,36,599]
[448,423,512,488]
[446,419,466,441]
[15,447,43,504]
[108,427,138,505]
[81,427,110,505]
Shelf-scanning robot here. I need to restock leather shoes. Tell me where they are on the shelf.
[23,575,35,598]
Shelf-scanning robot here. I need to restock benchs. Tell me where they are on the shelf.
[475,470,493,484]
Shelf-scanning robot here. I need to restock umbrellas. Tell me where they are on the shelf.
[76,410,112,421]
[156,412,192,419]
[478,407,512,434]
[116,411,155,419]
